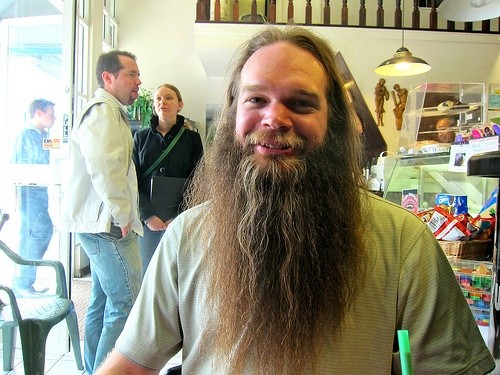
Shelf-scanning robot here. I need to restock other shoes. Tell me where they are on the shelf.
[10,276,49,298]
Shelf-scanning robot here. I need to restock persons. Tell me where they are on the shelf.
[93,28,495,375]
[10,100,56,298]
[436,118,458,144]
[373,78,389,126]
[132,84,206,281]
[391,83,408,130]
[59,50,145,375]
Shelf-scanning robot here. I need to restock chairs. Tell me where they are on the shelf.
[0,240,85,375]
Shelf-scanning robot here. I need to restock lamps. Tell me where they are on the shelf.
[374,0,432,77]
[438,0,500,22]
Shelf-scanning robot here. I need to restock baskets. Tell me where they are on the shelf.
[438,239,492,260]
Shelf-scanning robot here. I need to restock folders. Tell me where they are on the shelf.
[151,175,187,222]
